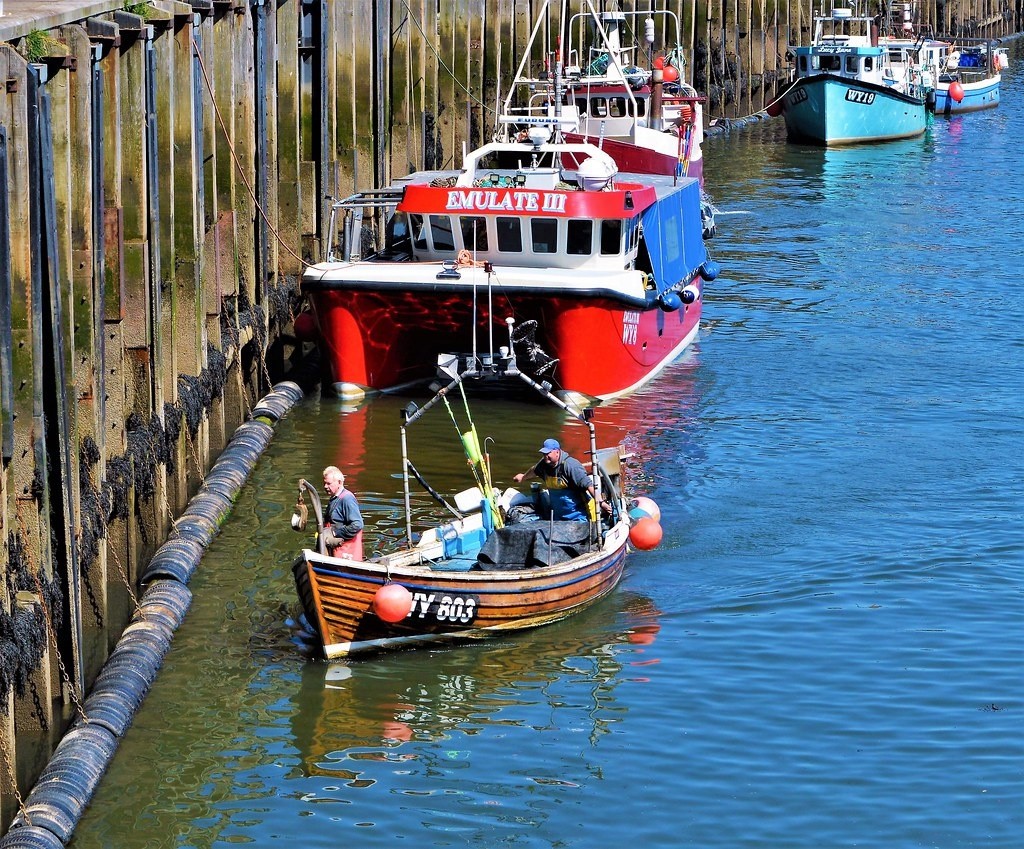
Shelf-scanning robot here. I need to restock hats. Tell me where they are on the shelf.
[537,439,561,454]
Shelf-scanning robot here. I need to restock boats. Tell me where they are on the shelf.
[772,6,929,146]
[930,32,1001,115]
[289,346,632,662]
[501,7,707,195]
[293,1,716,415]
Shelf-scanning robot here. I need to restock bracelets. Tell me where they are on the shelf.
[599,500,604,506]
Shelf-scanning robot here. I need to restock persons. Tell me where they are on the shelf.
[513,439,612,522]
[314,466,364,561]
